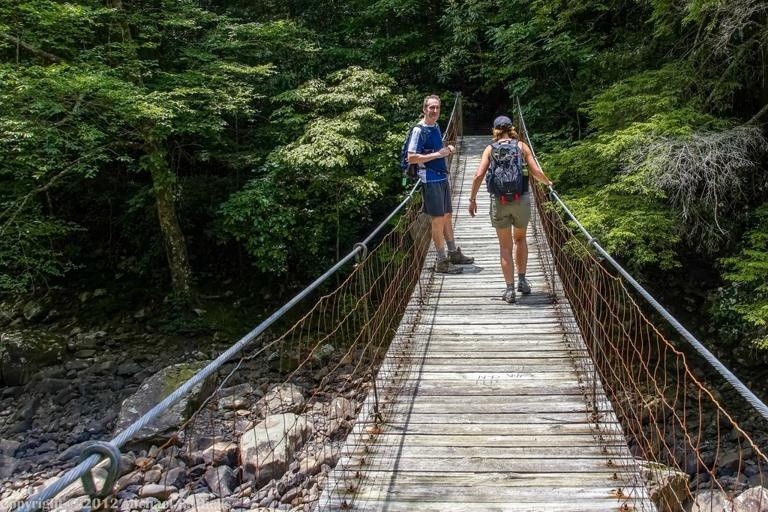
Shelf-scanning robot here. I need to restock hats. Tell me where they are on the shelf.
[493,115,512,129]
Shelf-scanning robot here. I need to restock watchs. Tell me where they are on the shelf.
[468,197,476,204]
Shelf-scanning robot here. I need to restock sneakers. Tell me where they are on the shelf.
[502,290,515,303]
[435,258,463,274]
[517,280,532,294]
[446,247,474,264]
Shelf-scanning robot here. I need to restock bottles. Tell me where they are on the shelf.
[522,162,530,178]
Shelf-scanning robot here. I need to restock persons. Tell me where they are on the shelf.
[467,114,553,305]
[403,94,475,276]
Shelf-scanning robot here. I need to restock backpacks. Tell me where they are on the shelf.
[399,123,435,180]
[484,138,529,195]
